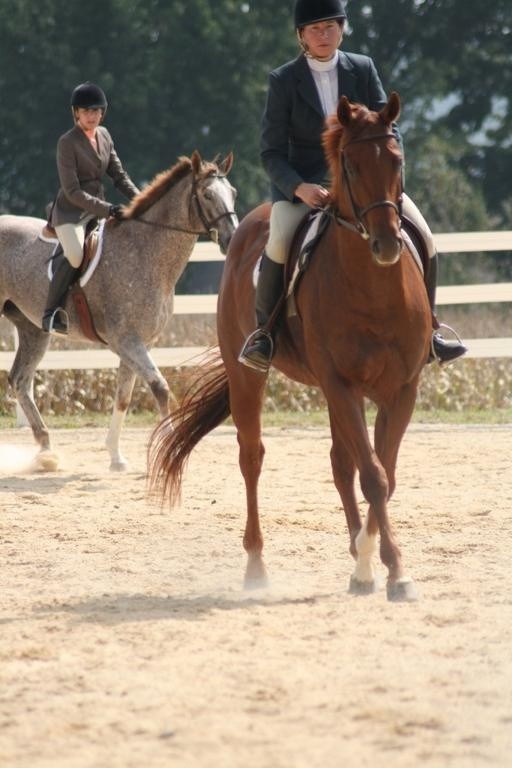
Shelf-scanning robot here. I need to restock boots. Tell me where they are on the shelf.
[42,256,80,332]
[243,249,291,366]
[424,246,469,368]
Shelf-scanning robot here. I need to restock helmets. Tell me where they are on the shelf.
[71,81,108,110]
[295,0,346,28]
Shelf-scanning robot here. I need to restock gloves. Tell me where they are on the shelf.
[110,204,126,219]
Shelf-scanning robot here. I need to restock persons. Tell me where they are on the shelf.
[244,1,468,369]
[40,79,141,333]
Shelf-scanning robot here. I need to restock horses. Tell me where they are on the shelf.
[144,92,437,607]
[0,145,243,475]
